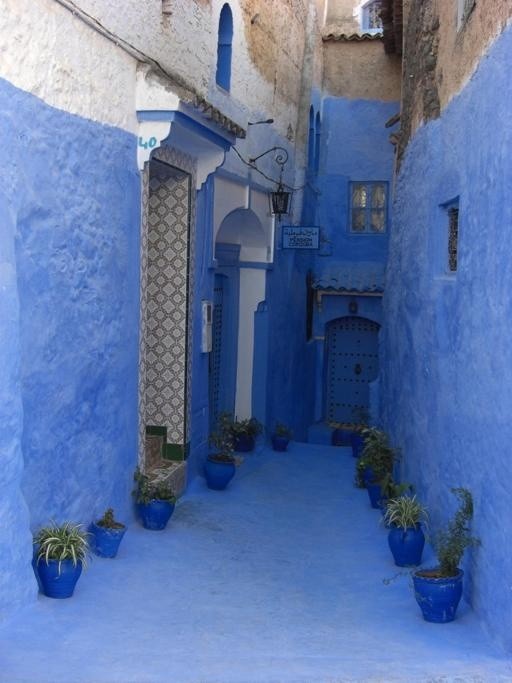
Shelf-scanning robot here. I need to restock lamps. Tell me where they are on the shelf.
[245,139,295,221]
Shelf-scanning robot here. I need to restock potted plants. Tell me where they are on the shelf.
[348,404,483,625]
[31,515,89,600]
[270,424,291,451]
[93,508,126,559]
[134,466,175,530]
[205,435,237,491]
[226,417,263,453]
